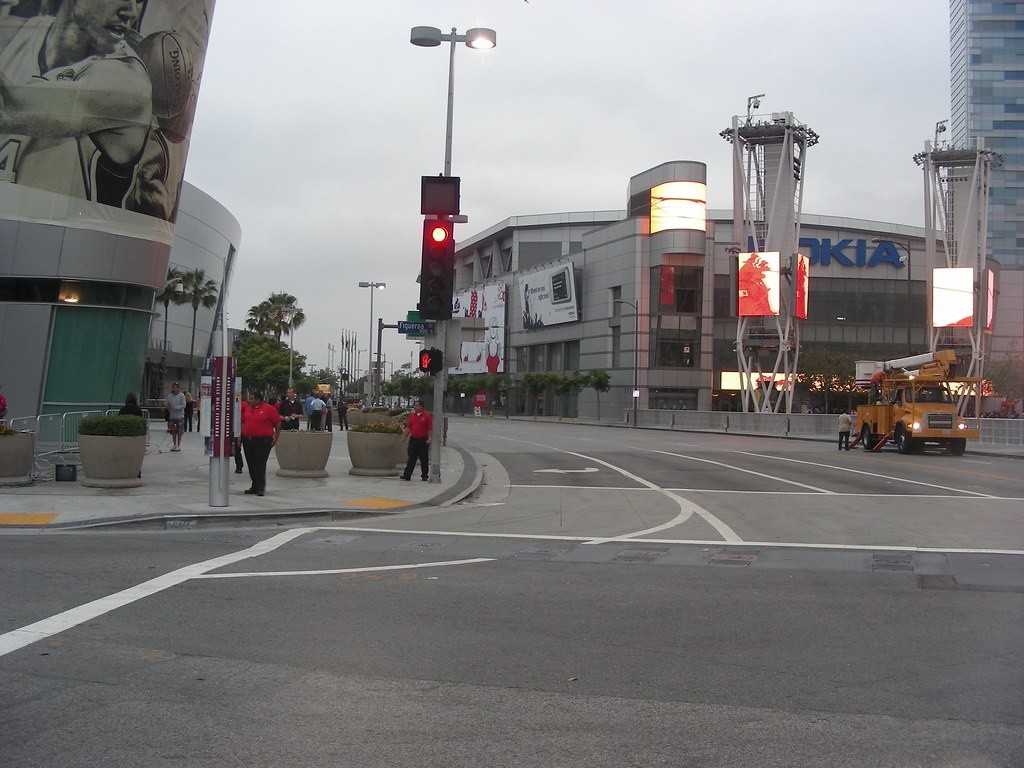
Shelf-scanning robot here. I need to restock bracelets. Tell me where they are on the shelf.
[427,438,431,440]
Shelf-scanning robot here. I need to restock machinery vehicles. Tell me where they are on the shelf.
[848,349,985,458]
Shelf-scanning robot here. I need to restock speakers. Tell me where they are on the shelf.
[55,464,77,482]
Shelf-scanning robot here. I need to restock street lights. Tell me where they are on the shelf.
[408,26,497,484]
[373,352,386,383]
[870,237,913,353]
[359,281,386,398]
[281,307,303,388]
[613,298,639,426]
[308,363,318,372]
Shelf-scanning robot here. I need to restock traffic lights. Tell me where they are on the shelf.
[418,346,443,377]
[417,219,456,321]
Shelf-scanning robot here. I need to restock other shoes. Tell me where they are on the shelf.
[346,428,348,431]
[256,491,263,496]
[175,446,181,451]
[235,470,242,473]
[839,448,841,450]
[845,448,849,450]
[245,489,254,494]
[171,446,175,451]
[422,477,427,481]
[400,475,411,480]
[340,429,343,430]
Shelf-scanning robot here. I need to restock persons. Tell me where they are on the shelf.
[871,369,891,405]
[119,393,141,479]
[166,383,186,451]
[233,391,280,496]
[267,387,348,433]
[805,406,825,414]
[838,410,851,450]
[400,400,432,481]
[0,386,7,418]
[165,390,201,433]
[0,0,153,208]
